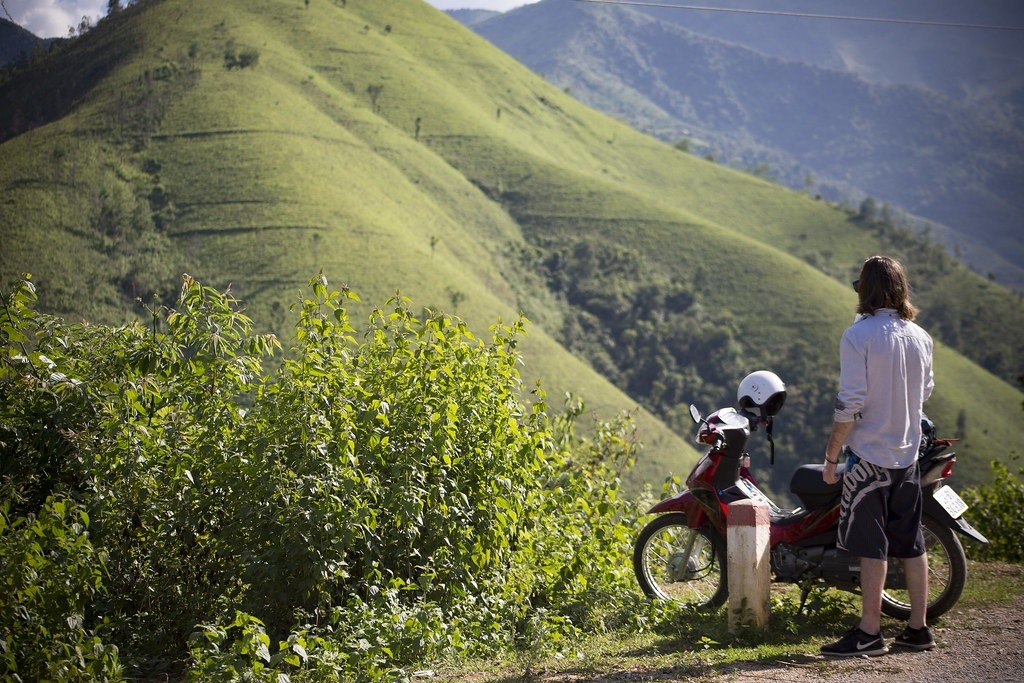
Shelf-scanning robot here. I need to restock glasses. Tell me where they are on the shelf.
[852,280,859,293]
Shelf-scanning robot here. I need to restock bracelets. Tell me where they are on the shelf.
[826,456,839,465]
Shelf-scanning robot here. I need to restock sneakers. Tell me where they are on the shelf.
[895,625,937,649]
[819,626,890,656]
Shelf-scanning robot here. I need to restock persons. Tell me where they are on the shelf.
[821,256,936,656]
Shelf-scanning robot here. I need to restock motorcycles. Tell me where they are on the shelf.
[631,404,991,623]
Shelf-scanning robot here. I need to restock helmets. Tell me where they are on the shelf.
[737,370,786,416]
